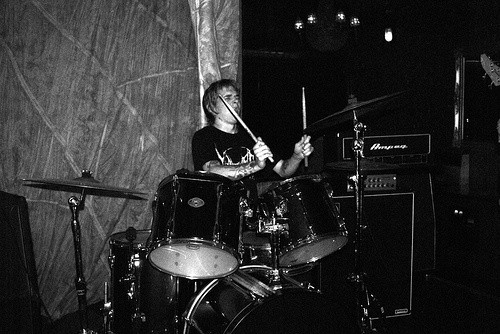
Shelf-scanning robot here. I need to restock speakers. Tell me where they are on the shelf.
[317,191,412,320]
[0,190,43,334]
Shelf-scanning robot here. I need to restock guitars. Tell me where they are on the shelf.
[478,53,500,89]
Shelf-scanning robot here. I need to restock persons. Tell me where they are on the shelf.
[191,78,314,182]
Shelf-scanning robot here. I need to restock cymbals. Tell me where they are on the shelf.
[301,87,415,137]
[20,175,151,197]
[324,158,407,172]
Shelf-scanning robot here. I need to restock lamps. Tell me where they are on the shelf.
[384,28,393,41]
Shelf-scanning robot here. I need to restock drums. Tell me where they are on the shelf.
[238,230,315,275]
[248,170,350,270]
[146,170,244,281]
[104,228,197,333]
[177,264,367,333]
